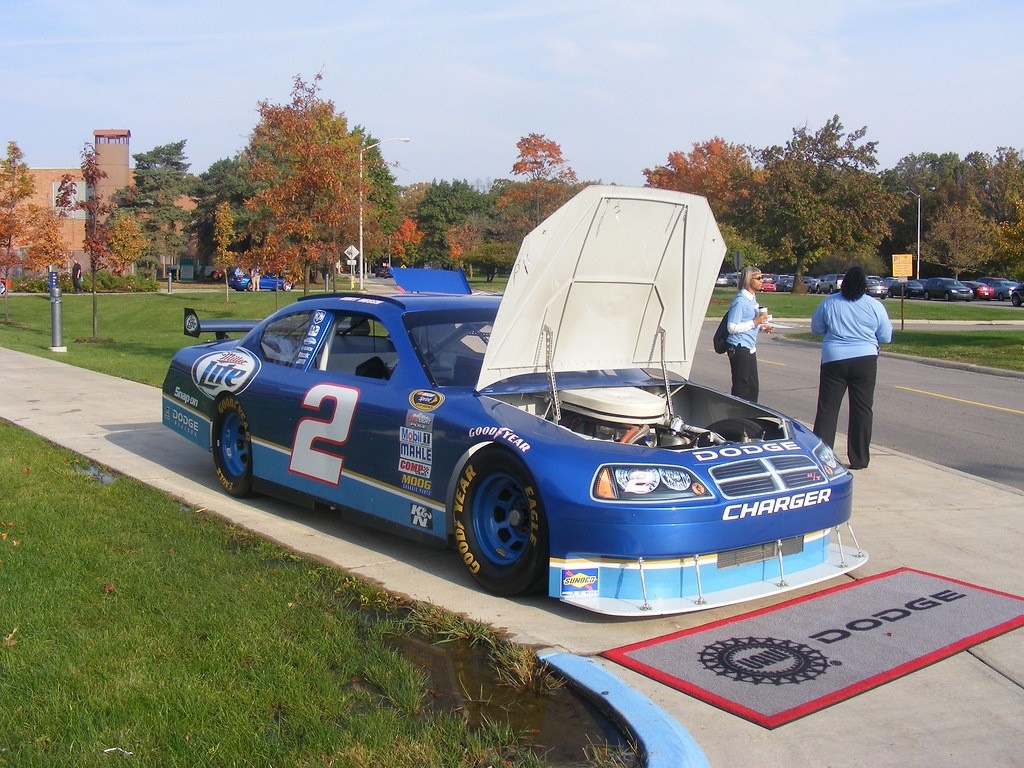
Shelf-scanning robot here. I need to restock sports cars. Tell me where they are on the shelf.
[158,182,871,618]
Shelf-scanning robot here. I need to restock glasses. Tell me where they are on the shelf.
[751,277,763,281]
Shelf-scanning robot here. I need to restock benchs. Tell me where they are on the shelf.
[275,336,401,378]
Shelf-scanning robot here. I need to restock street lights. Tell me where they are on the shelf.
[355,136,411,288]
[902,184,937,281]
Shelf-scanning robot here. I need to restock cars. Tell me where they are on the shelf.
[990,280,1021,301]
[865,279,889,300]
[976,277,1008,283]
[913,278,927,285]
[959,280,995,301]
[0,276,11,293]
[923,277,974,303]
[1011,282,1024,306]
[888,280,924,299]
[713,270,898,294]
[228,261,296,292]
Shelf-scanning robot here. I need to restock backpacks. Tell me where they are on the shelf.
[714,296,743,354]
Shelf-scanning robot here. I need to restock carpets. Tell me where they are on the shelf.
[596,567,1024,730]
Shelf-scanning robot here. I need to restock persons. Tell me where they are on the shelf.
[72,258,83,294]
[810,266,892,469]
[725,266,774,403]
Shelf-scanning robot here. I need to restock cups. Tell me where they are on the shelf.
[758,306,768,319]
